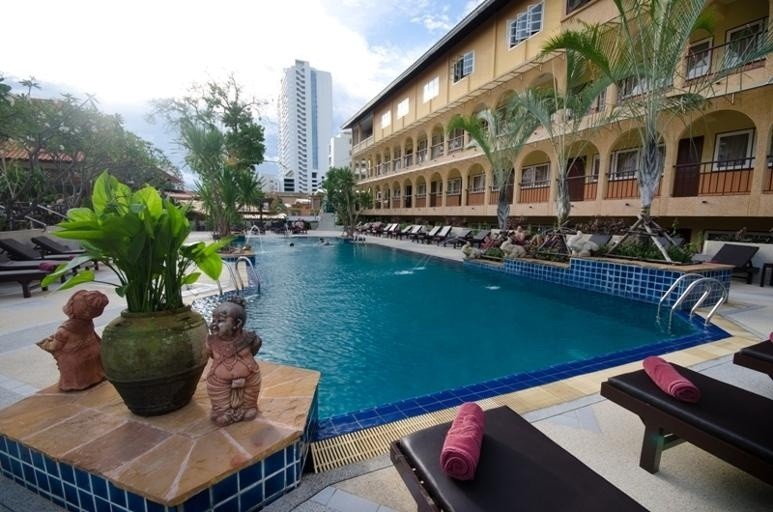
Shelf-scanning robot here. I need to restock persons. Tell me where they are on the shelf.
[527,234,551,250]
[511,225,525,246]
[202,295,262,427]
[319,237,330,247]
[38,290,109,391]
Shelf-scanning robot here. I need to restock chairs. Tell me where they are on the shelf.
[357,221,453,247]
[0,235,100,298]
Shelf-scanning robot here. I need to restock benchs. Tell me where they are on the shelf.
[599,360,772,488]
[390,403,648,511]
[732,338,772,377]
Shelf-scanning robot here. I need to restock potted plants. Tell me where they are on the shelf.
[40,169,242,417]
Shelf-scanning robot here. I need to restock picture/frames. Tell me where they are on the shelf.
[591,142,665,183]
[710,126,755,172]
[685,36,716,80]
[416,161,550,200]
[724,15,765,70]
